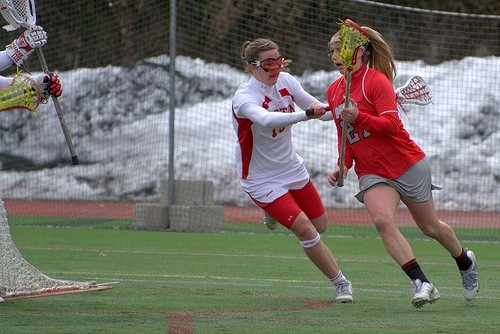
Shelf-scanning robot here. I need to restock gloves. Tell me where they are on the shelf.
[38,73,62,98]
[5,26,48,67]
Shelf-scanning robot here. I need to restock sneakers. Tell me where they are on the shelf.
[410,279,441,307]
[335,279,353,302]
[263,212,277,230]
[458,250,480,300]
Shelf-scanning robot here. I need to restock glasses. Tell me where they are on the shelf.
[260,55,284,72]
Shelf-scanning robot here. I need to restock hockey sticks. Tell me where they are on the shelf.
[0,0,80,166]
[305,75,435,117]
[0,73,41,113]
[336,18,371,188]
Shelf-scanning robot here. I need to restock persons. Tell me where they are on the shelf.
[326,26,481,309]
[230,37,353,304]
[0,25,63,101]
